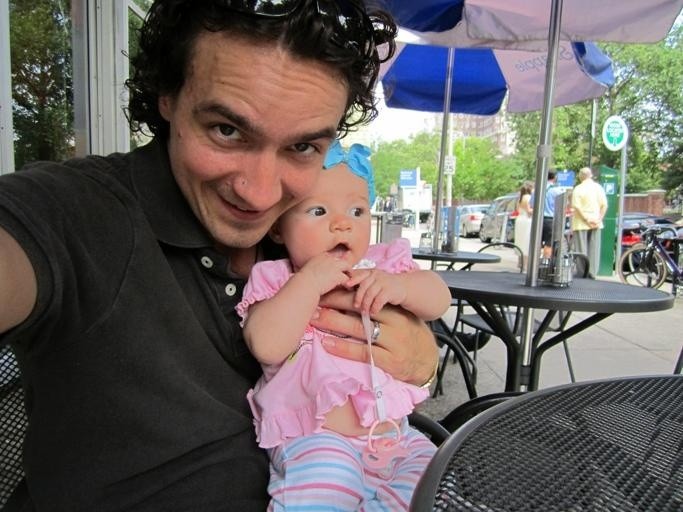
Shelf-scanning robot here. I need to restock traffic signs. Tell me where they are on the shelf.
[441,155,456,175]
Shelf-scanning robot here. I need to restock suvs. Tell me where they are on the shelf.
[478,195,520,247]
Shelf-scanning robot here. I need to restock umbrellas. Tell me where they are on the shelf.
[369,0,683,393]
[376,36,615,271]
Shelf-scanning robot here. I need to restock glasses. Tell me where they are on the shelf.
[245,0,377,59]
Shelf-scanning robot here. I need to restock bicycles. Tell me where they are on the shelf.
[611,216,681,298]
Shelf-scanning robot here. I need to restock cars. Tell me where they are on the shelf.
[618,212,683,273]
[370,184,434,224]
[458,203,492,239]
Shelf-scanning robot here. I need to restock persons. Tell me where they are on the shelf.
[527,167,566,259]
[0,0,397,510]
[513,178,533,274]
[231,138,453,511]
[568,165,609,280]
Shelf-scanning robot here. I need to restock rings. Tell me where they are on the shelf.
[370,321,382,343]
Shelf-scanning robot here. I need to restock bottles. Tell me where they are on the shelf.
[375,199,397,213]
[418,232,454,254]
[538,257,575,288]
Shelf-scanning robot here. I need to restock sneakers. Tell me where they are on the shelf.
[458,331,490,351]
